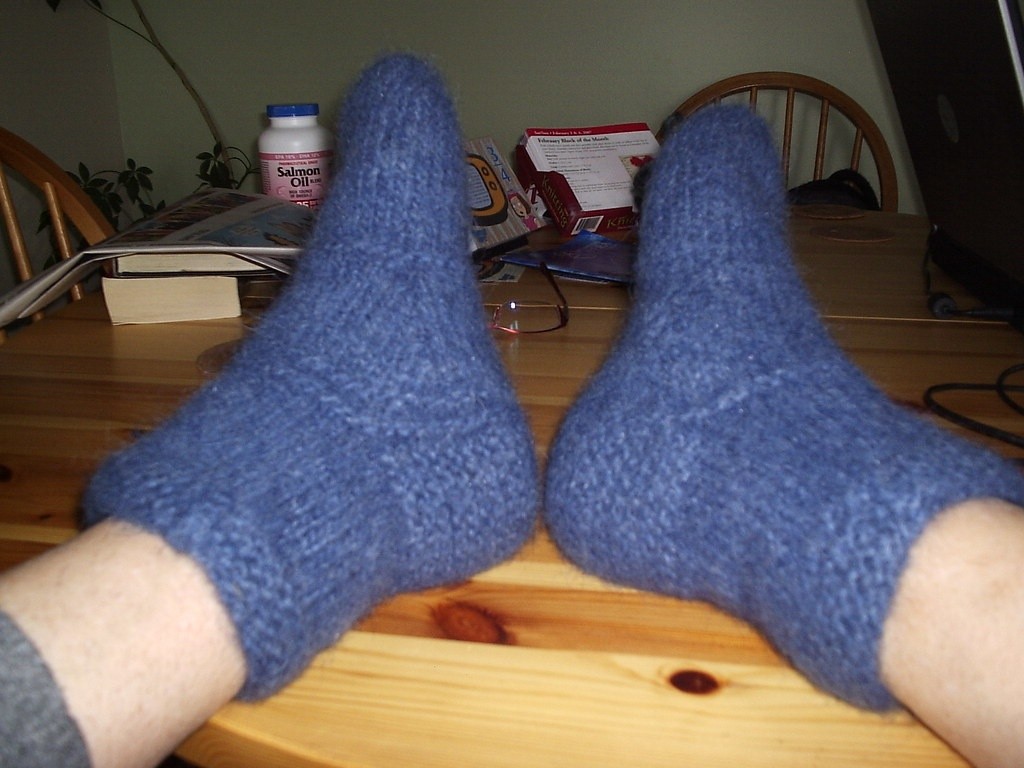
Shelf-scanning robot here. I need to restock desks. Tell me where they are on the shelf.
[0,204,1024,768]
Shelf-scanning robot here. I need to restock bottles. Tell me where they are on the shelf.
[258,103,332,215]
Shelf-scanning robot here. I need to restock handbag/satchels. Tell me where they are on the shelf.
[787,169,879,211]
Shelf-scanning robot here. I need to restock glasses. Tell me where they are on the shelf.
[487,260,568,334]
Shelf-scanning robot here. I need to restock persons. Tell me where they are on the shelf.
[0,52,1024,768]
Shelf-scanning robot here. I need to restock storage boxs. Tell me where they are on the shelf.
[517,123,661,238]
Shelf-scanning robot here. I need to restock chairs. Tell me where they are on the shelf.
[0,127,118,344]
[655,71,899,212]
